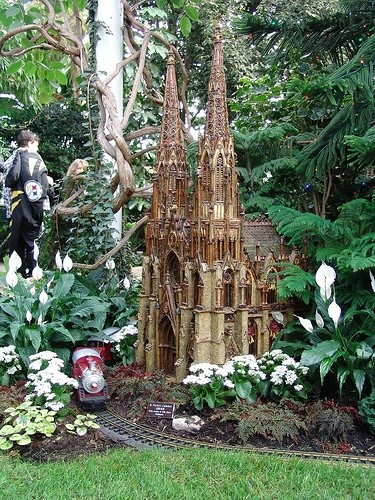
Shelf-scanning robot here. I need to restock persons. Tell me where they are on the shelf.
[51,158,91,273]
[3,130,57,279]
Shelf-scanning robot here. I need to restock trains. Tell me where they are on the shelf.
[72,326,121,411]
